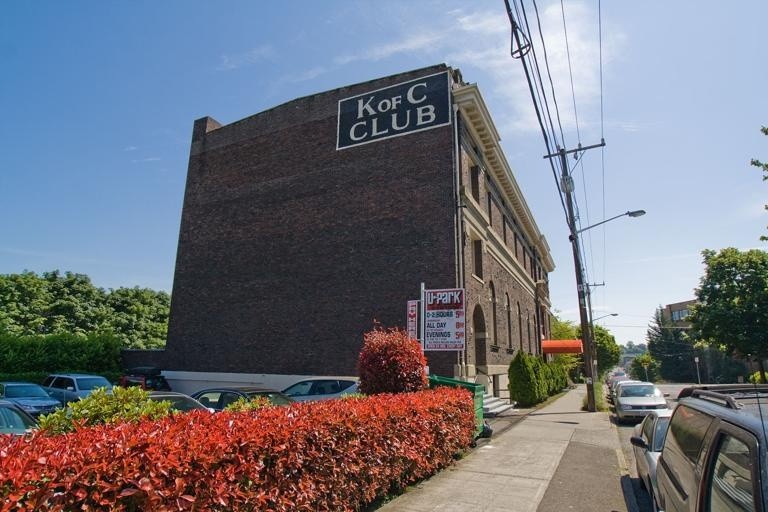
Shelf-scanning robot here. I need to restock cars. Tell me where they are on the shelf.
[602,367,766,510]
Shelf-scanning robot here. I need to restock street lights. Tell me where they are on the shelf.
[694,355,703,381]
[585,313,620,325]
[565,209,647,413]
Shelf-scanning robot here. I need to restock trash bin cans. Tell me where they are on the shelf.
[428,376,493,447]
